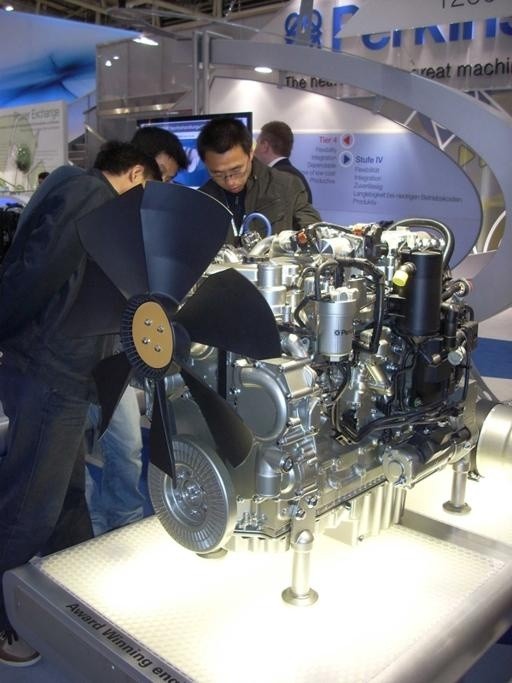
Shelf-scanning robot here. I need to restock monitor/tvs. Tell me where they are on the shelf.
[136,111,253,190]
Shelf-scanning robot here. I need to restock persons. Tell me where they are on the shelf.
[197,112,321,249]
[0,136,160,668]
[253,119,312,230]
[84,125,188,536]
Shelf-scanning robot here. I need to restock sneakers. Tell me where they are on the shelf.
[0,631,42,669]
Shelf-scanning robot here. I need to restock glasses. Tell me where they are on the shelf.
[209,156,250,180]
